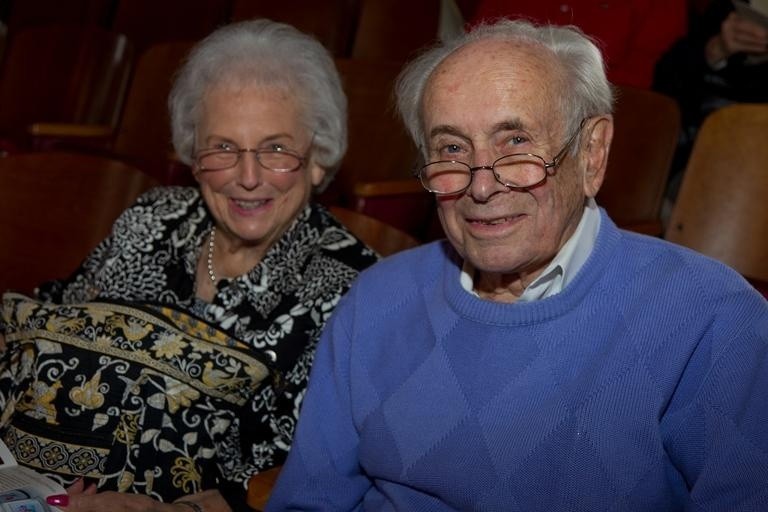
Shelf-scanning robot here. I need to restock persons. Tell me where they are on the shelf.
[650,0,767,234]
[263,15,768,511]
[0,19,390,511]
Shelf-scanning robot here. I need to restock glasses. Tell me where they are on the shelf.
[411,117,587,194]
[191,126,315,173]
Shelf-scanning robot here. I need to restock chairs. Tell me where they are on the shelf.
[0,1,766,511]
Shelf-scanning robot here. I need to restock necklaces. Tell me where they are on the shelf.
[207,223,218,286]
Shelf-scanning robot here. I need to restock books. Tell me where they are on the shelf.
[0,439,69,511]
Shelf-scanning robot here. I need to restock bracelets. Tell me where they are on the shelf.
[174,498,201,511]
